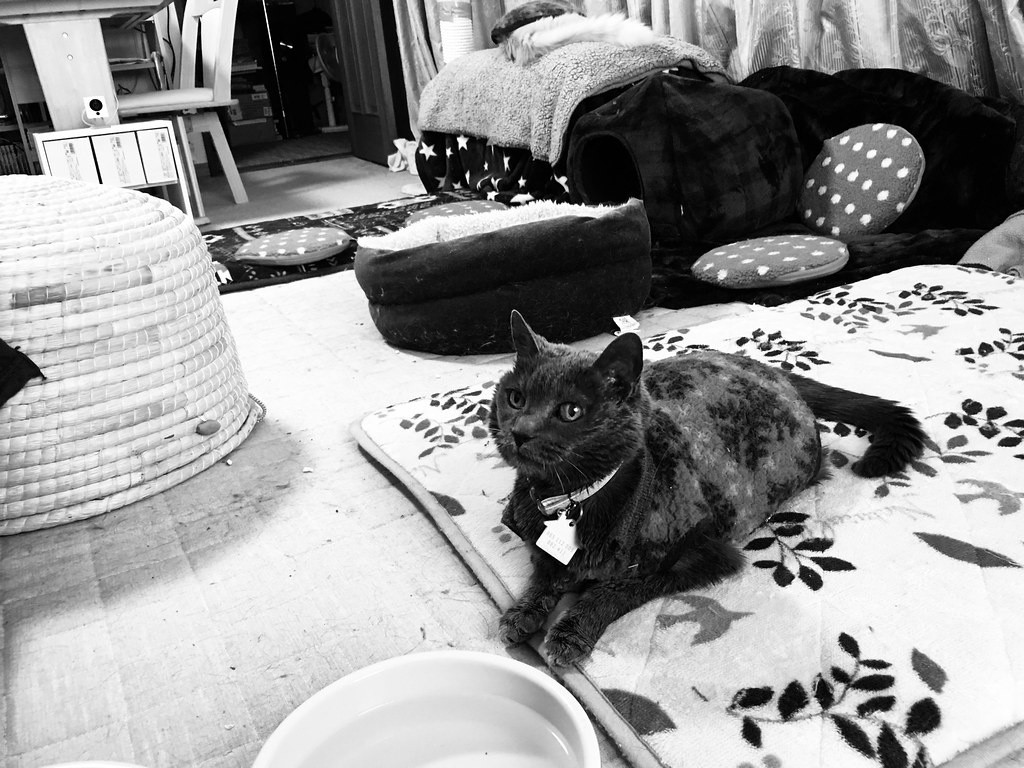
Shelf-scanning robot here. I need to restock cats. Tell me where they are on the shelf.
[487,306,928,670]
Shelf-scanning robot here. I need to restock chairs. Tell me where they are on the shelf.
[116,0,248,214]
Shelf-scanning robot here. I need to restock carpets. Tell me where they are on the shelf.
[355,263,1024,768]
[200,193,448,295]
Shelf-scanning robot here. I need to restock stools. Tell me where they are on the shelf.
[416,43,740,206]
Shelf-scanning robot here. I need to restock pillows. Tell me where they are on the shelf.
[237,228,350,267]
[692,233,850,286]
[406,199,508,224]
[797,123,926,239]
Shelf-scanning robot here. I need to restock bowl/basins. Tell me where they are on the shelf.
[249,650,603,768]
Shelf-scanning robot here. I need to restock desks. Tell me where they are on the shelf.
[0,0,209,229]
